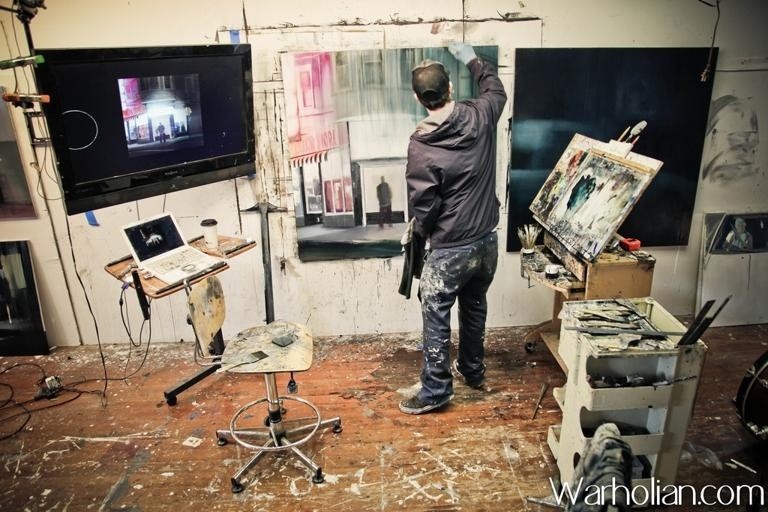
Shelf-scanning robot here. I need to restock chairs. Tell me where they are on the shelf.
[186,274,341,493]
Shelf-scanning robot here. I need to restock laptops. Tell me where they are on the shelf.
[122,214,227,287]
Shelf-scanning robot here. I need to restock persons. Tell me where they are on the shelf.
[396,38,509,414]
[723,218,753,250]
[376,175,393,228]
[156,122,165,143]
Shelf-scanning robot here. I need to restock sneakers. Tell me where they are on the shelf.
[453,360,486,388]
[398,393,454,415]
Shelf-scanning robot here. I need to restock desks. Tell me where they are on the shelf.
[104,232,256,405]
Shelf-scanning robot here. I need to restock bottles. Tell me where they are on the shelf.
[521,246,535,280]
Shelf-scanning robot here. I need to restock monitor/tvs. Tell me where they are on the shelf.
[28,43,255,217]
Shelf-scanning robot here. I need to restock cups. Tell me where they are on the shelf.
[200,219,220,251]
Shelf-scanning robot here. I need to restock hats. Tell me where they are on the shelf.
[411,58,451,108]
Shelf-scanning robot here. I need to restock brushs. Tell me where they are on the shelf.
[216,351,269,373]
[625,120,647,143]
[576,305,646,329]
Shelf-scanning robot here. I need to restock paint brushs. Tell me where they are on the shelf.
[617,126,630,141]
[531,382,549,420]
[632,135,641,144]
[517,223,542,249]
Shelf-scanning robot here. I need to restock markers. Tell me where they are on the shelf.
[2,93,50,103]
[0,55,45,69]
